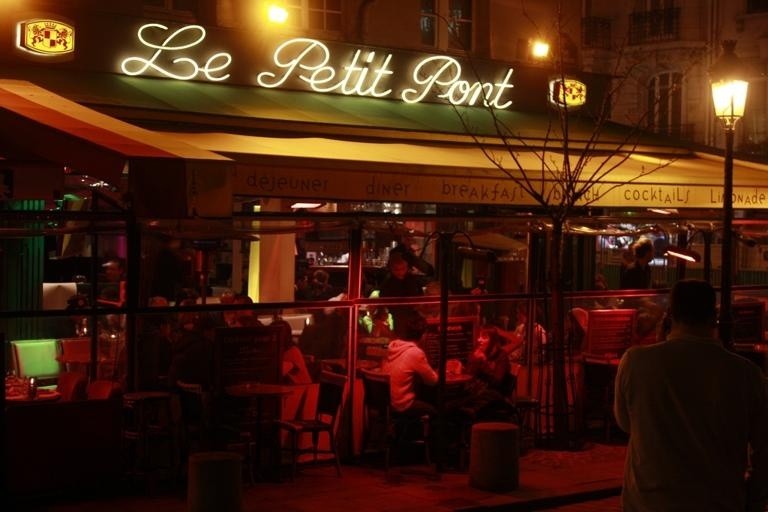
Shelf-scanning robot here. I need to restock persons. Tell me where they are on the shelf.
[381,311,440,437]
[618,237,656,289]
[504,302,548,363]
[465,326,511,415]
[96,257,126,309]
[365,244,436,333]
[307,269,335,298]
[223,293,263,328]
[265,318,312,384]
[137,296,211,383]
[295,272,308,298]
[613,278,768,512]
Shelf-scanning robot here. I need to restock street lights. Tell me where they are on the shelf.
[696,29,758,357]
